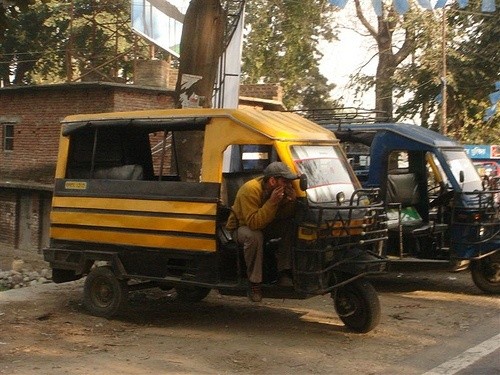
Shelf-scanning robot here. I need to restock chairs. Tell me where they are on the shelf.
[388,175,449,255]
[221,172,264,279]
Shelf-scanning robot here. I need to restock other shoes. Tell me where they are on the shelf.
[276,273,293,287]
[247,283,263,302]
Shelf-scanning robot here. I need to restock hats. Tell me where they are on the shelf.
[263,160,299,180]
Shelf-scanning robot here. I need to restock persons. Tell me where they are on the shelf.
[225,162,301,302]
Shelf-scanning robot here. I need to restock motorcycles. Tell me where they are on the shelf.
[40,106,390,334]
[291,105,500,293]
